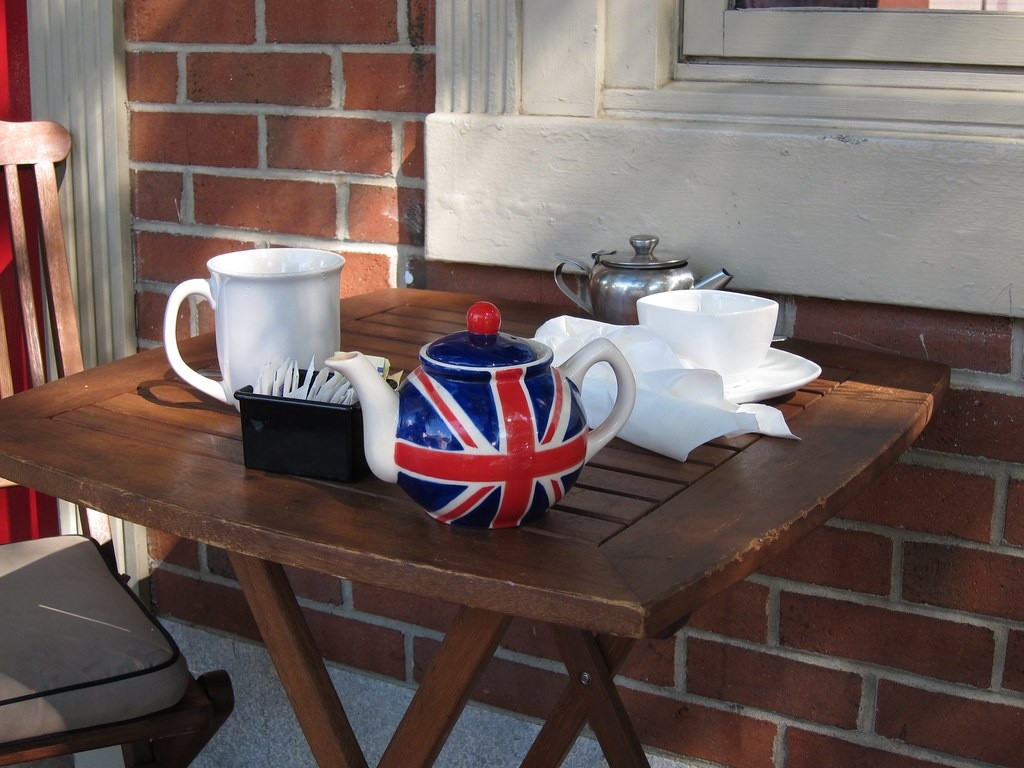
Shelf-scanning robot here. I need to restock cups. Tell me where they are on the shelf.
[162,247,346,413]
[636,288,780,381]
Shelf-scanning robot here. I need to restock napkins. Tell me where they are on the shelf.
[527,314,802,461]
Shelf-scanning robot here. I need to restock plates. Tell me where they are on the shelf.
[612,325,823,404]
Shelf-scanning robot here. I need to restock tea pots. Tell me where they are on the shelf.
[325,301,637,530]
[554,235,734,328]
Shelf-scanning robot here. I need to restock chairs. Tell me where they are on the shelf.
[0,120,235,768]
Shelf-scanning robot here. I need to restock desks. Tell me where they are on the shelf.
[0,288,951,768]
